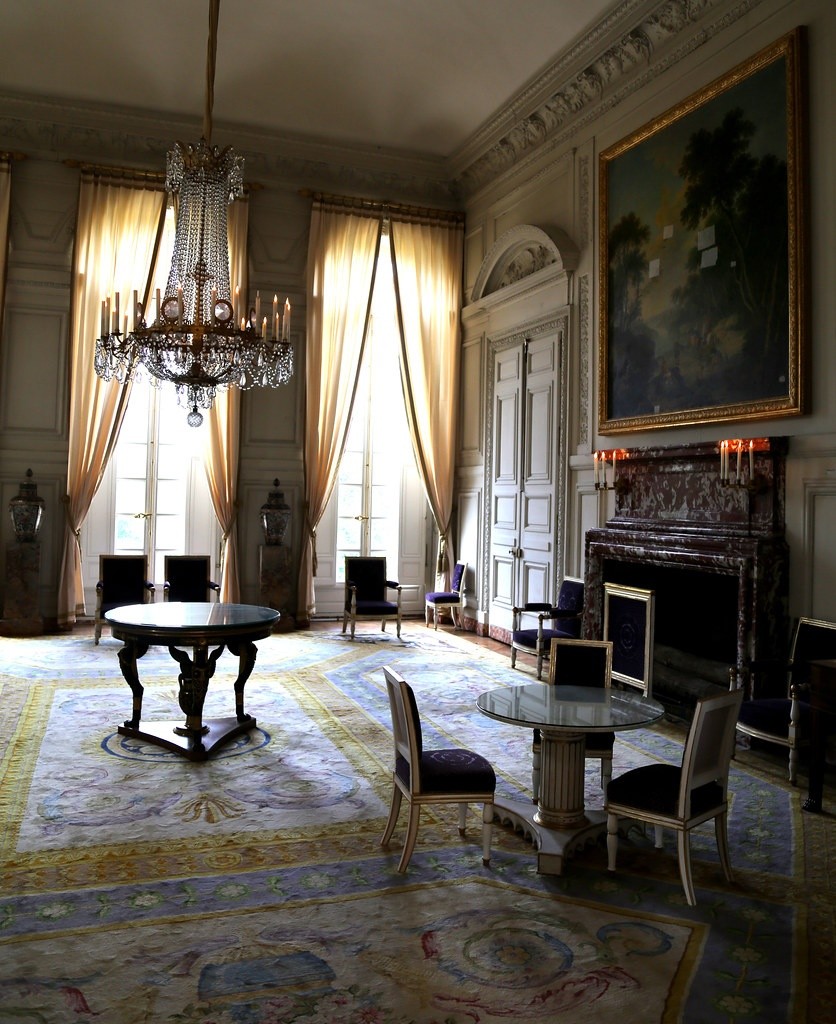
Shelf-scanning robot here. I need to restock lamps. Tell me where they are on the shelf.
[94,0,295,428]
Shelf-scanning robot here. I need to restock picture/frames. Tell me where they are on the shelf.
[596,26,808,437]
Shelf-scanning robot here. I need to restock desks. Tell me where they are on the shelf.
[476,683,666,877]
[104,601,281,763]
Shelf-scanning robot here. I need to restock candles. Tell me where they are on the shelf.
[749,440,754,481]
[736,442,742,480]
[602,452,606,483]
[720,441,724,480]
[725,440,730,479]
[612,451,617,482]
[594,452,599,483]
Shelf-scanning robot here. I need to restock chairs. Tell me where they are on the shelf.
[602,688,744,908]
[164,555,221,602]
[530,637,616,810]
[343,555,402,639]
[380,664,496,875]
[94,554,156,646]
[511,575,584,680]
[425,560,469,631]
